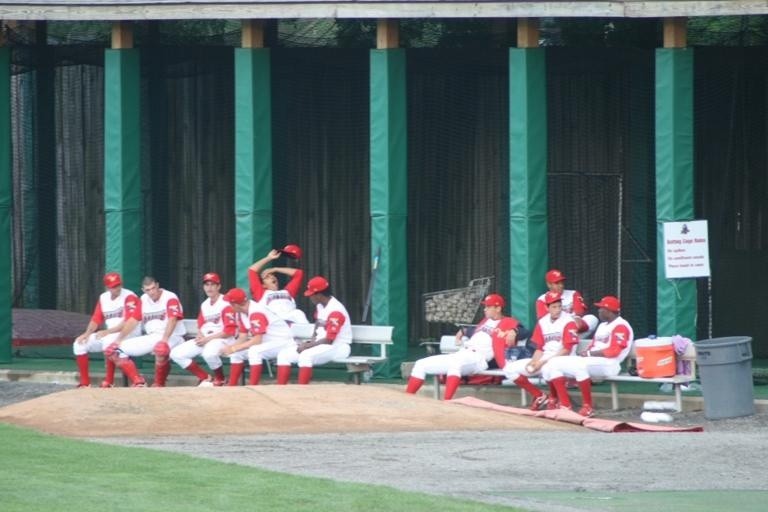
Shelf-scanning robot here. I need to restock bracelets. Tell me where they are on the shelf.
[230,345,236,352]
[511,328,519,334]
[461,329,465,335]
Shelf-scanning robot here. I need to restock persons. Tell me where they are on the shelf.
[535,268,599,339]
[72,271,138,387]
[219,287,295,385]
[248,247,309,328]
[168,271,239,386]
[546,295,634,419]
[103,274,184,387]
[275,275,353,385]
[502,290,579,411]
[405,292,530,401]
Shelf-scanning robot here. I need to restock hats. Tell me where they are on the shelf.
[102,273,123,288]
[303,275,330,297]
[202,272,221,285]
[479,293,506,308]
[277,242,304,260]
[544,268,567,284]
[544,289,565,305]
[222,287,248,304]
[592,294,622,313]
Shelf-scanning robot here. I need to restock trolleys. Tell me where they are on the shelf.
[420,274,498,356]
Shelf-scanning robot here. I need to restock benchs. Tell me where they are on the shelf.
[123,318,394,387]
[433,337,696,413]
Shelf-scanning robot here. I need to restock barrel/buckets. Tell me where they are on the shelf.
[633,338,676,380]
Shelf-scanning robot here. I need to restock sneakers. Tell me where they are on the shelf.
[130,381,148,389]
[578,401,594,418]
[560,404,572,411]
[197,373,226,386]
[99,380,115,388]
[546,397,557,409]
[530,391,550,410]
[75,382,92,388]
